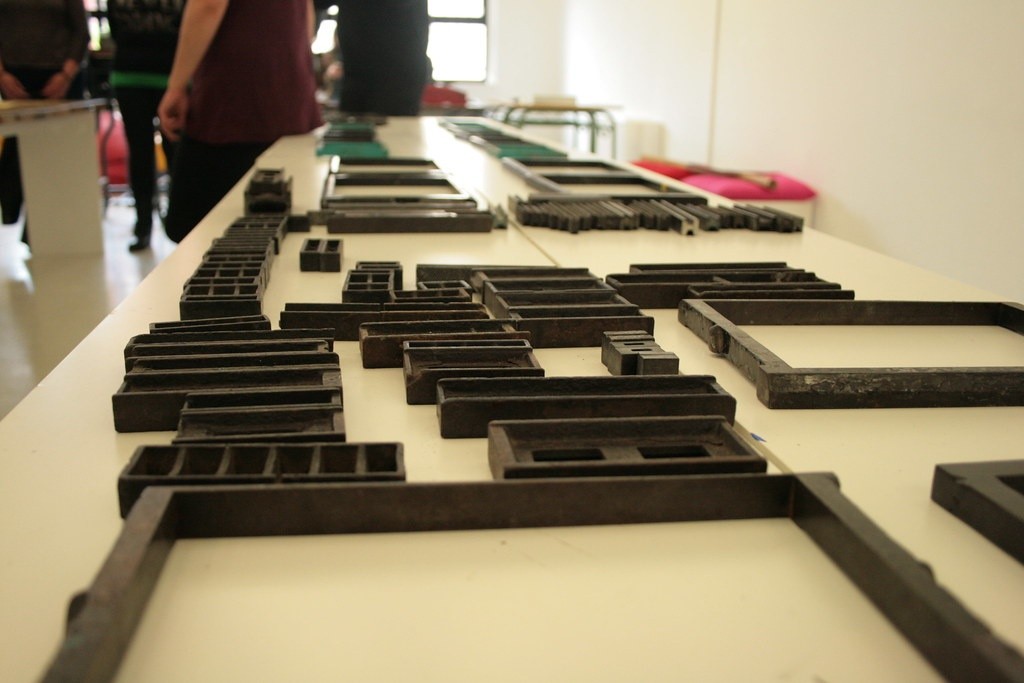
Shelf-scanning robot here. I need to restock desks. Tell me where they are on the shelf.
[1,114,1023,681]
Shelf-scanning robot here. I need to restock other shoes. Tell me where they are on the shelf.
[129,233,151,251]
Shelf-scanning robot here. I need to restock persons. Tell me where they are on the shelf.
[318,0,428,118]
[0,0,91,257]
[108,0,186,252]
[158,0,324,244]
